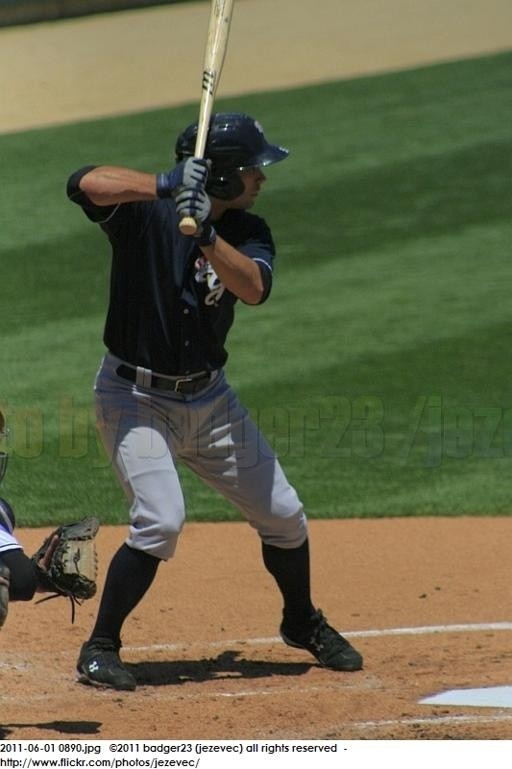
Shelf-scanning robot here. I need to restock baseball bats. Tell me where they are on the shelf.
[179,0,235,237]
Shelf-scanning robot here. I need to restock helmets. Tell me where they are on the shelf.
[176,112,289,200]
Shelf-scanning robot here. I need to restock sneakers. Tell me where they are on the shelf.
[76,640,136,691]
[280,608,362,671]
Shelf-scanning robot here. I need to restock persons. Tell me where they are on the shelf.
[0,410,100,630]
[67,113,362,692]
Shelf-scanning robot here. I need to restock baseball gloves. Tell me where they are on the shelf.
[34,513,101,622]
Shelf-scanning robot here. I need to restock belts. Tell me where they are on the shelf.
[116,365,211,393]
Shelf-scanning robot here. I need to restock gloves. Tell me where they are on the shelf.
[157,157,215,247]
